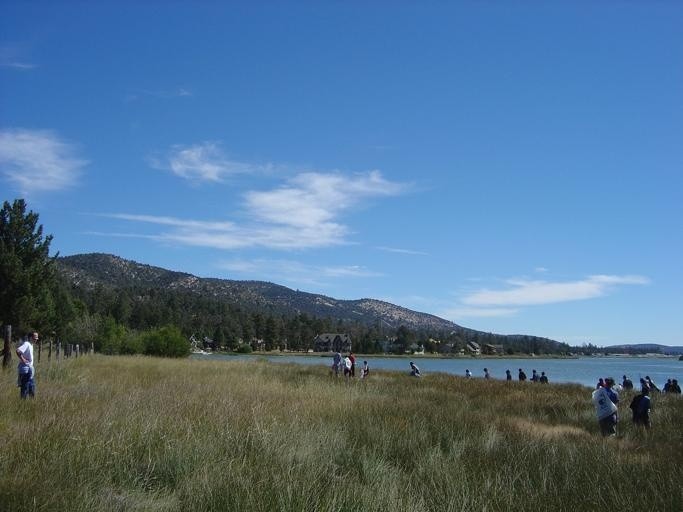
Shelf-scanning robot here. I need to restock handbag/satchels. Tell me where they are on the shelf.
[592,388,617,419]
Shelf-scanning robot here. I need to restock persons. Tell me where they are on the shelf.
[410,362,420,377]
[506,369,512,380]
[630,387,652,429]
[596,379,620,436]
[518,368,526,381]
[465,369,472,378]
[484,368,489,378]
[597,375,681,395]
[540,372,548,384]
[530,369,540,382]
[334,351,355,378]
[363,360,369,379]
[16,330,39,401]
